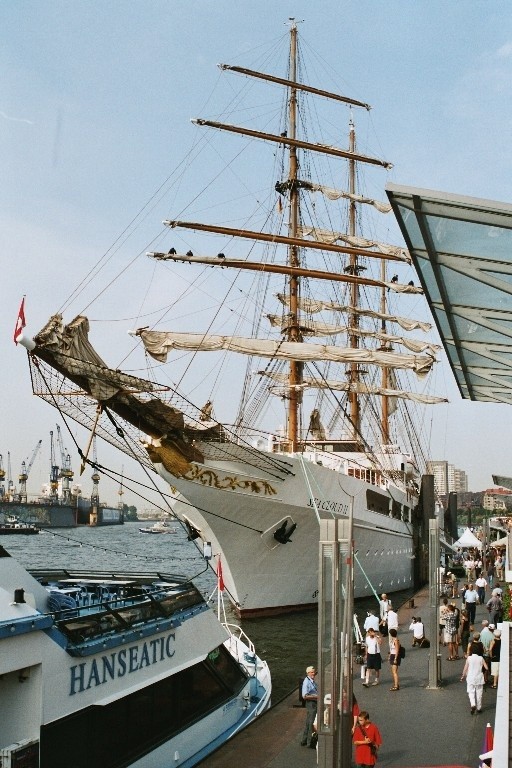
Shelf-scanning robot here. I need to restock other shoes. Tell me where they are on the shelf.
[471,706,477,715]
[372,681,379,686]
[476,710,482,714]
[471,620,475,624]
[382,634,386,637]
[301,742,307,746]
[412,641,416,647]
[362,682,370,688]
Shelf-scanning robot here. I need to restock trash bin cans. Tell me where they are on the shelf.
[427,654,441,685]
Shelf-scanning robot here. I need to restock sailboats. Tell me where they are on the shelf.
[15,16,452,621]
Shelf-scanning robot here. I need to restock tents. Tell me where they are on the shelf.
[452,527,507,557]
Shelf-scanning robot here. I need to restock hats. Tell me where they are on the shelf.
[490,591,498,596]
[488,624,496,629]
[447,572,452,575]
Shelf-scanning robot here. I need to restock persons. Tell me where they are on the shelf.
[353,711,382,768]
[313,694,331,768]
[301,666,318,749]
[435,547,503,715]
[362,593,405,690]
[338,686,359,734]
[409,616,425,647]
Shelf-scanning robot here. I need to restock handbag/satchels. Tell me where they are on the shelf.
[371,745,377,755]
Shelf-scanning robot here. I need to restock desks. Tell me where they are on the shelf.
[152,582,180,588]
[58,579,137,608]
[48,590,79,597]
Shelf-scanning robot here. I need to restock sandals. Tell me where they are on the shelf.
[462,655,468,659]
[489,684,497,689]
[390,687,399,691]
[454,656,460,659]
[446,657,455,661]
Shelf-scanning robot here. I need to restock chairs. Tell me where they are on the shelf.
[46,582,184,618]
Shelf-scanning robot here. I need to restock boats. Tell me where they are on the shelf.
[447,526,484,578]
[0,421,273,768]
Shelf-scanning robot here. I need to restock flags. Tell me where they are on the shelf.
[217,554,225,591]
[13,296,27,346]
